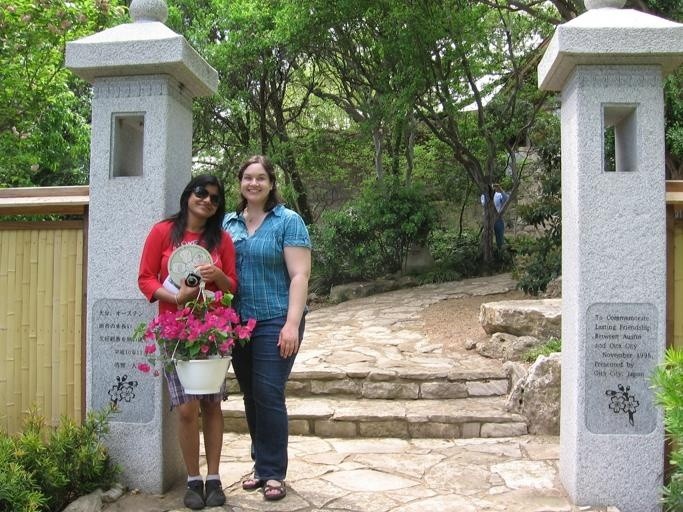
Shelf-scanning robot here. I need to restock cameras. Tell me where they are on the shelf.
[492,184,499,188]
[184,272,201,288]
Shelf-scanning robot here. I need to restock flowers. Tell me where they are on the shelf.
[129,290,258,377]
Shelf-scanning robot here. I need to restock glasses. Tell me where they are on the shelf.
[191,186,221,207]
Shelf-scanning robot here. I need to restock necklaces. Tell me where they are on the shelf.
[245,209,260,223]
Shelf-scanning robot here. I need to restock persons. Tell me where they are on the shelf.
[220,153,312,502]
[138,173,237,511]
[479,181,507,251]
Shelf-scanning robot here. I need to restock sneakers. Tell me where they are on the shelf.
[203,479,226,507]
[183,478,205,510]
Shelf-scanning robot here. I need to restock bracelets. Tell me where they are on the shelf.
[174,293,180,307]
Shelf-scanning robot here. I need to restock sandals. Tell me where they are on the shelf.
[262,477,287,501]
[240,465,263,491]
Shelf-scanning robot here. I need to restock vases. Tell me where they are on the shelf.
[173,356,232,397]
